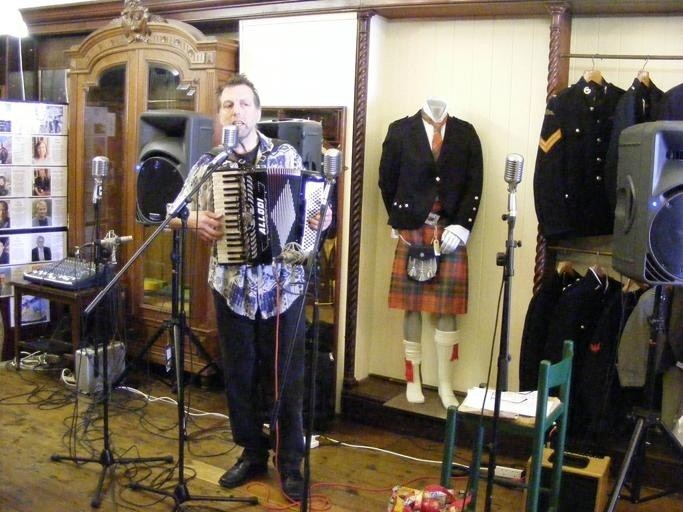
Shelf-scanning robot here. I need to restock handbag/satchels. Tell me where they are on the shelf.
[388,486,471,512]
[406,244,440,281]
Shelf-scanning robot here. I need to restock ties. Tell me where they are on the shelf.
[423,113,448,161]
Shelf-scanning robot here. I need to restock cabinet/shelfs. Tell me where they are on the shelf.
[61,1,240,391]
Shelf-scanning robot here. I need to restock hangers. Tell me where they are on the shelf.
[552,246,644,295]
[580,53,651,89]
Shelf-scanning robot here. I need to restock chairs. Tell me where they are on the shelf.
[441,339,574,511]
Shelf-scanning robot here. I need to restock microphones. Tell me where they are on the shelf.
[325,148,341,183]
[503,153,524,192]
[169,217,182,255]
[272,252,309,266]
[222,124,237,150]
[90,156,109,189]
[92,235,133,247]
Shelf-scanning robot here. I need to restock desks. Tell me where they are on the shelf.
[6,278,130,373]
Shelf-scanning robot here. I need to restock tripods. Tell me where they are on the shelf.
[95,229,225,439]
[130,218,258,512]
[605,286,683,512]
[50,265,173,509]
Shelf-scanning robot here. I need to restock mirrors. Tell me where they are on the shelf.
[80,59,200,319]
[259,106,348,367]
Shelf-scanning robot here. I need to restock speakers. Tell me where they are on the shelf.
[612,121,683,285]
[135,110,214,224]
[258,118,322,172]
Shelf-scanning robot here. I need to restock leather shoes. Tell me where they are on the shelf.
[219,449,269,486]
[273,457,303,502]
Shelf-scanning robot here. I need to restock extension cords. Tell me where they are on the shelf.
[303,436,319,449]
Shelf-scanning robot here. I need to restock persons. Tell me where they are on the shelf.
[165,71,333,506]
[34,142,47,160]
[0,200,9,230]
[30,236,51,261]
[0,240,8,265]
[32,199,51,227]
[34,170,50,196]
[0,142,8,164]
[375,93,485,412]
[0,174,8,196]
[0,273,10,295]
[42,108,61,132]
